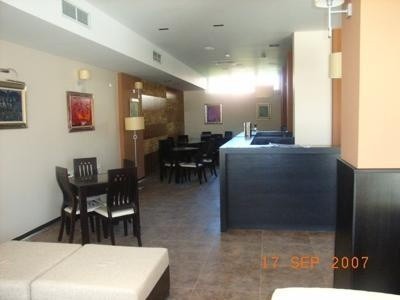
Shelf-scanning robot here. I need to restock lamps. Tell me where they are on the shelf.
[124,116,145,190]
[79,70,91,81]
[134,82,143,98]
[314,0,352,38]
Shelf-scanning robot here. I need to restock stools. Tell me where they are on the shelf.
[31,240,171,300]
[0,241,81,300]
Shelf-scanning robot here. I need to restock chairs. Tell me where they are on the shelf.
[158,131,233,184]
[55,157,143,247]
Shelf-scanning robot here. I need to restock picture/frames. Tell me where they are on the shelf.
[203,104,223,124]
[0,81,28,130]
[66,91,95,132]
[255,103,271,120]
